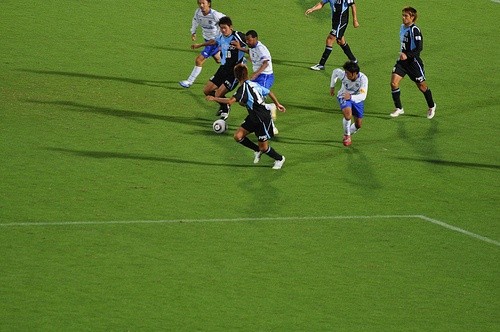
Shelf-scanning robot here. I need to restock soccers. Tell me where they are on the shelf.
[212,118,227,134]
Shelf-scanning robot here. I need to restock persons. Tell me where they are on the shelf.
[230,30,279,135]
[330,61,368,146]
[204,64,286,170]
[179,0,227,88]
[389,7,437,119]
[190,14,251,121]
[304,0,360,70]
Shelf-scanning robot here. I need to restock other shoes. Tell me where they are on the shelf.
[427,103,437,119]
[272,156,285,169]
[253,151,262,164]
[216,104,231,121]
[179,80,189,88]
[343,135,352,146]
[273,127,279,134]
[390,107,404,117]
[310,64,324,71]
[270,103,277,121]
